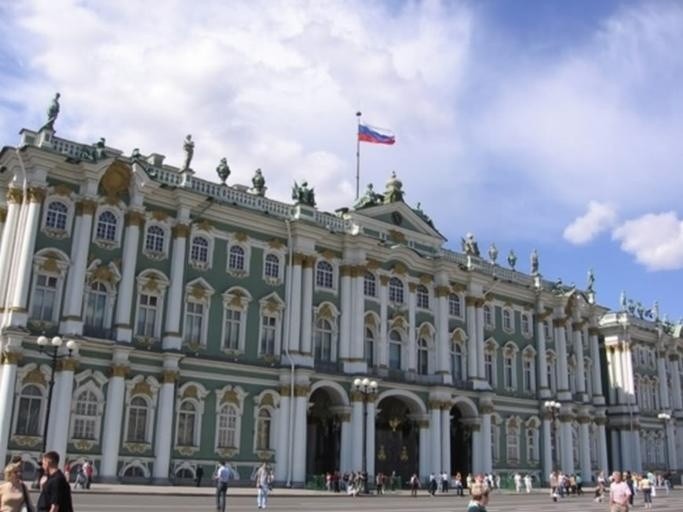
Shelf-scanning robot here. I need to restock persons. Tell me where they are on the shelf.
[587,267,595,291]
[216,461,232,512]
[291,179,316,207]
[39,92,61,133]
[196,464,204,486]
[620,286,660,325]
[462,233,481,258]
[0,451,93,512]
[530,247,539,274]
[183,134,195,172]
[364,183,385,203]
[256,462,271,509]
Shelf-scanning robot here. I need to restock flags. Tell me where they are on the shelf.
[358,120,395,145]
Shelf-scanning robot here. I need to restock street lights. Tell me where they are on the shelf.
[352,378,378,494]
[544,400,561,477]
[34,335,78,487]
[655,412,673,487]
